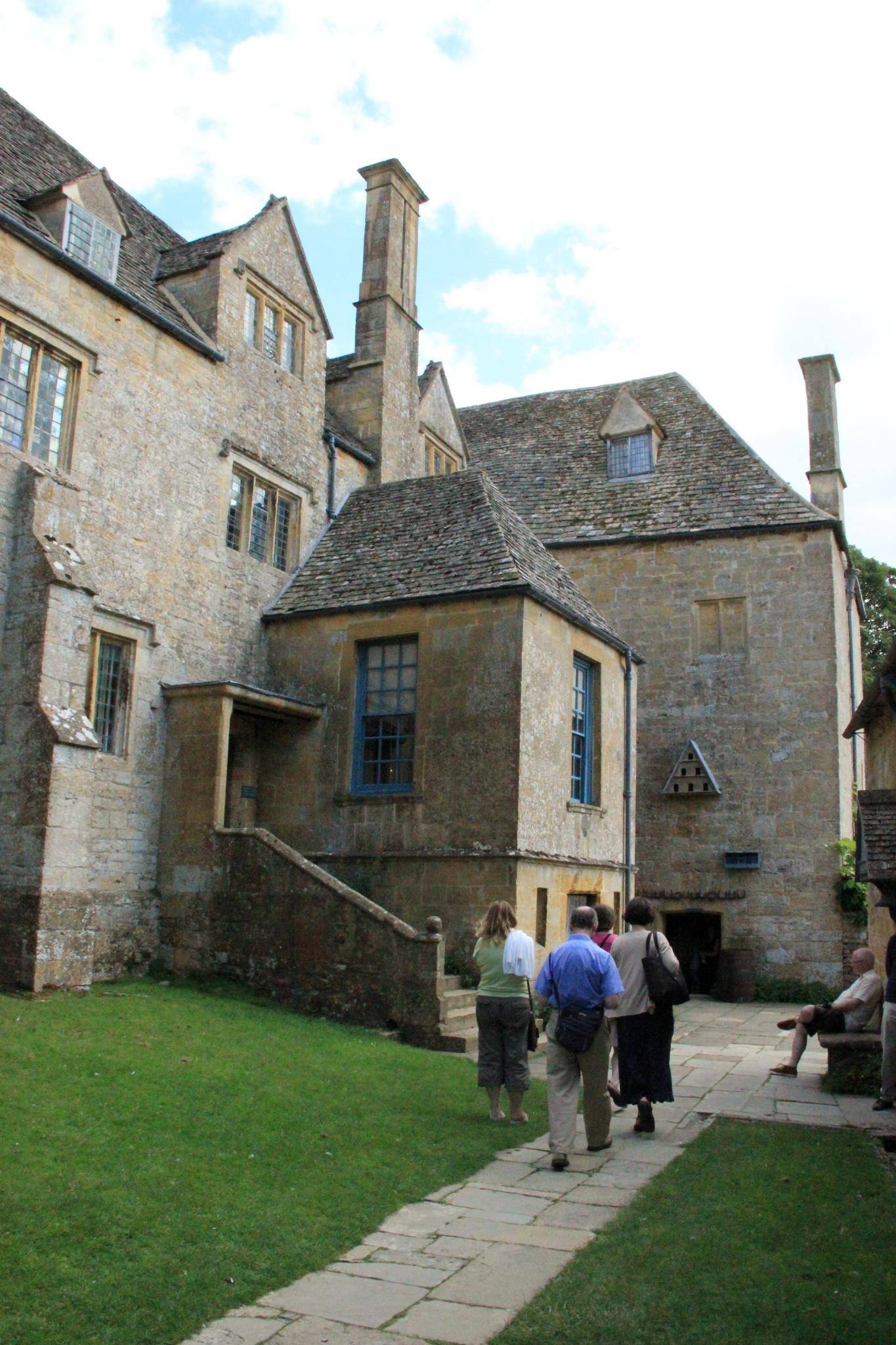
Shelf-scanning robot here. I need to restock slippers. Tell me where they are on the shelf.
[490,1109,506,1120]
[510,1112,530,1124]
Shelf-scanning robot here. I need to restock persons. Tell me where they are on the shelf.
[769,908,896,1110]
[534,897,682,1166]
[472,900,533,1125]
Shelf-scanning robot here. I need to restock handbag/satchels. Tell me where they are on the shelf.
[527,1013,539,1052]
[641,931,691,1008]
[555,1005,602,1053]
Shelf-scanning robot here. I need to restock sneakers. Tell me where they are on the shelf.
[770,1063,798,1077]
[777,1018,797,1030]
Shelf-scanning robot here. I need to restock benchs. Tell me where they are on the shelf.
[818,1031,882,1074]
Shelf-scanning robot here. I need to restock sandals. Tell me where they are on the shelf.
[607,1080,627,1108]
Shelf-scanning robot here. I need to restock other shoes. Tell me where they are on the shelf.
[872,1099,893,1110]
[634,1099,655,1132]
[586,1136,613,1151]
[551,1152,569,1167]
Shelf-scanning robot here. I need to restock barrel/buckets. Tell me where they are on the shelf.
[720,948,756,1003]
